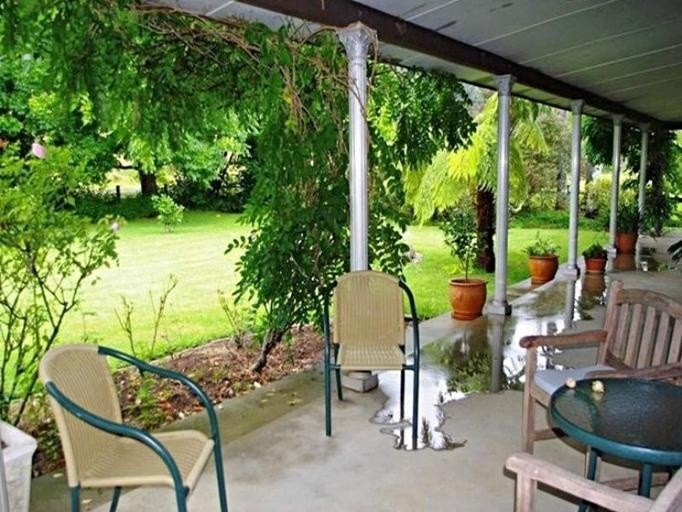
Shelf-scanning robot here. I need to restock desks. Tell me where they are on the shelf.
[548,376,682,512]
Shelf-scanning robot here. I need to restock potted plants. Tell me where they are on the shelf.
[439,199,494,324]
[581,203,645,273]
[517,233,563,283]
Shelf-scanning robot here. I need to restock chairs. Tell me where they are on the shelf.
[323,269,420,439]
[39,342,228,512]
[518,280,682,491]
